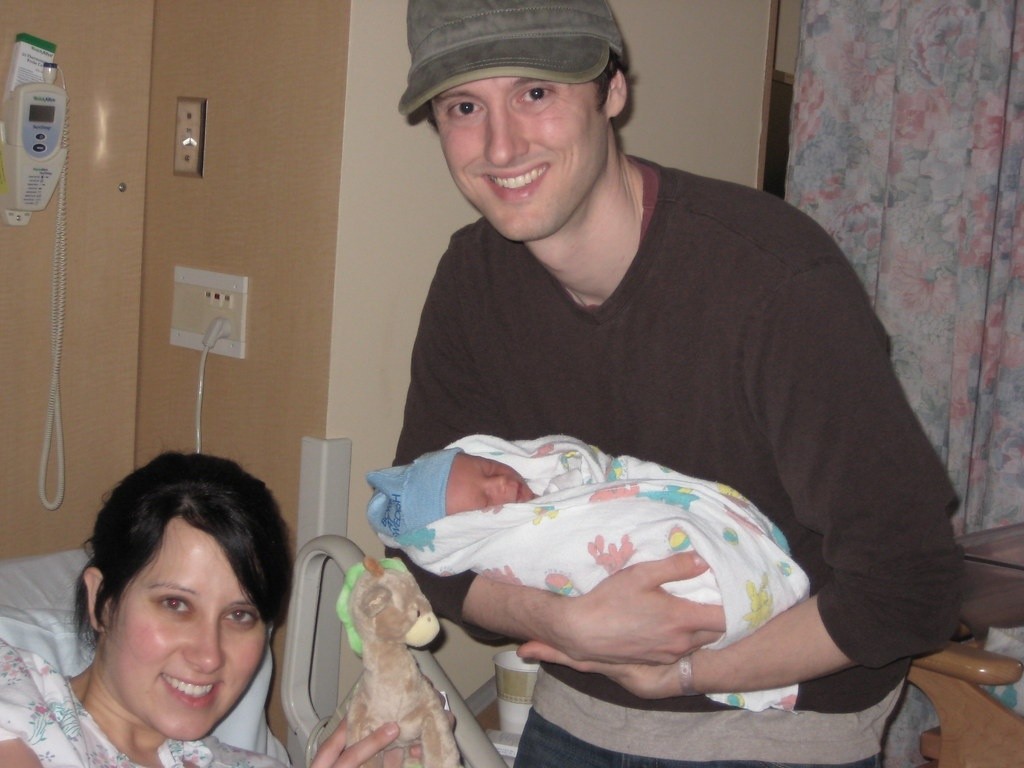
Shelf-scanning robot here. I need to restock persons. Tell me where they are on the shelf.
[364,436,810,713]
[0,452,456,768]
[386,0,960,768]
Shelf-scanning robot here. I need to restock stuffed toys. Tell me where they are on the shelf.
[334,555,461,768]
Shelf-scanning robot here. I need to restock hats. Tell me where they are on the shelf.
[366,446,466,538]
[397,0,625,116]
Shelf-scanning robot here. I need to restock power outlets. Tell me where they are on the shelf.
[168,264,249,359]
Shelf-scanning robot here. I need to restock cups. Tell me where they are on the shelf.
[493,651,540,735]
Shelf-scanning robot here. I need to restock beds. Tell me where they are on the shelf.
[0,435,516,768]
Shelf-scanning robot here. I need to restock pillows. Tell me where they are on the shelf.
[0,602,275,758]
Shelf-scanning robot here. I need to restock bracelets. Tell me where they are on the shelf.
[677,654,699,697]
